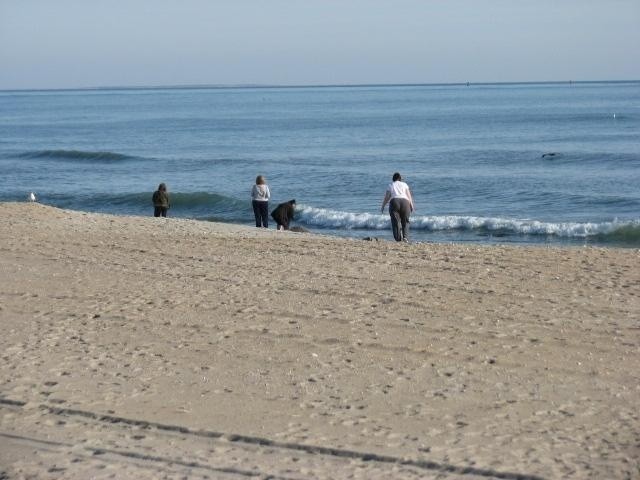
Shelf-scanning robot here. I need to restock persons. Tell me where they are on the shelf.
[270,199,296,230]
[381,172,413,242]
[152,183,170,217]
[251,175,270,228]
